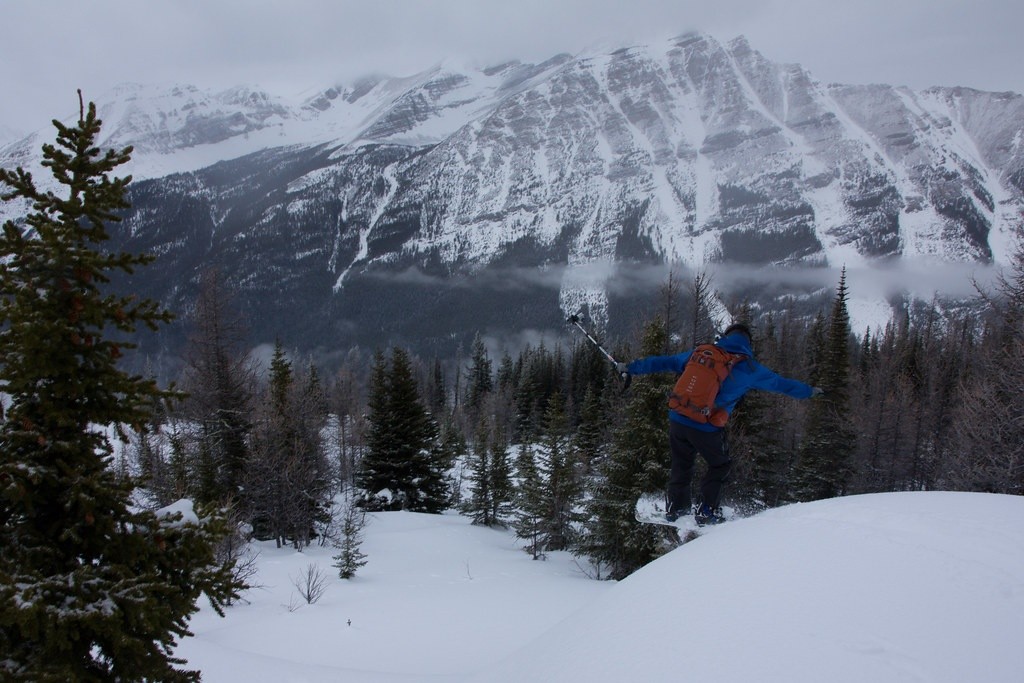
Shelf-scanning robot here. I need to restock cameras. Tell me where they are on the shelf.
[576,312,584,323]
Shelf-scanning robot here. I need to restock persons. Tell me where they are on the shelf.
[616,324,823,528]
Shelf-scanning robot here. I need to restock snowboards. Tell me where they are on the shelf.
[635,511,726,531]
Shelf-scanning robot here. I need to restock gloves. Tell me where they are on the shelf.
[810,387,827,398]
[615,362,628,373]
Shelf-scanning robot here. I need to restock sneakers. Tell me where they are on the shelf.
[695,508,724,525]
[665,509,691,521]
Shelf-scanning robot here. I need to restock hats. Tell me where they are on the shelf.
[724,324,751,344]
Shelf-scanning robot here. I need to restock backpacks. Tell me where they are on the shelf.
[668,344,748,427]
